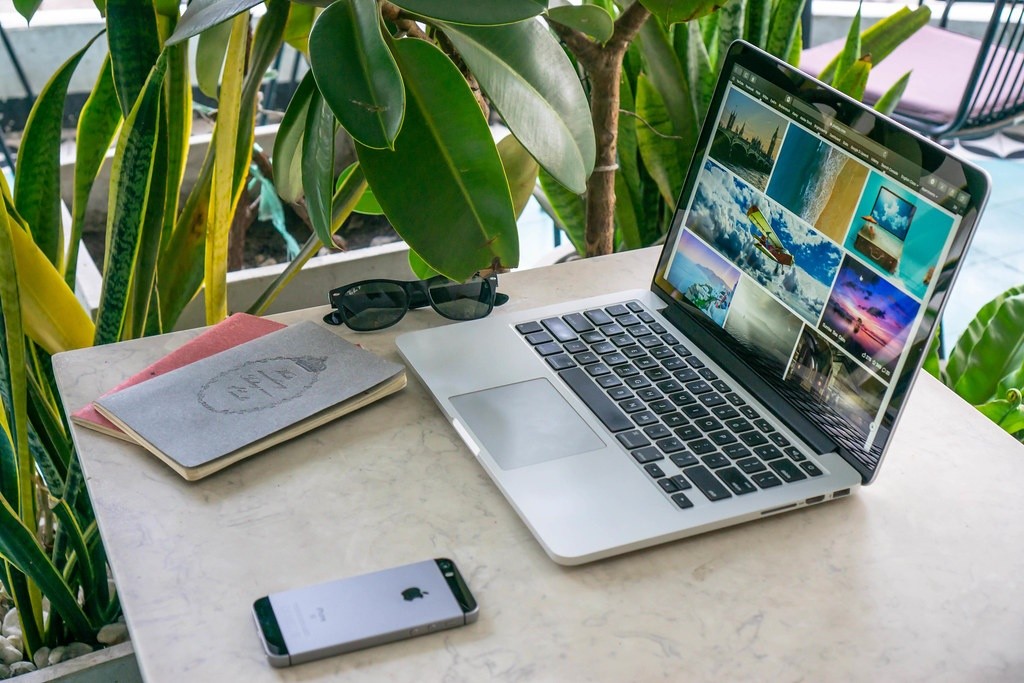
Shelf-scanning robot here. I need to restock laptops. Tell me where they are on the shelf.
[396,41,994,566]
[858,186,917,260]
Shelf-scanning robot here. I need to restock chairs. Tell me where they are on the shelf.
[796,0,1024,149]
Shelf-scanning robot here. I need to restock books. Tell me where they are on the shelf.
[70,312,408,482]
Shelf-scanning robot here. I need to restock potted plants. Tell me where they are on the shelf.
[0,0,506,683]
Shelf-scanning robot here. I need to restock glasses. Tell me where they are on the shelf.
[323,273,509,332]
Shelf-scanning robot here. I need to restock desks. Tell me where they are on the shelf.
[50,246,1024,683]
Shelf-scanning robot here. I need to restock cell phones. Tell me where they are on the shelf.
[251,558,479,668]
